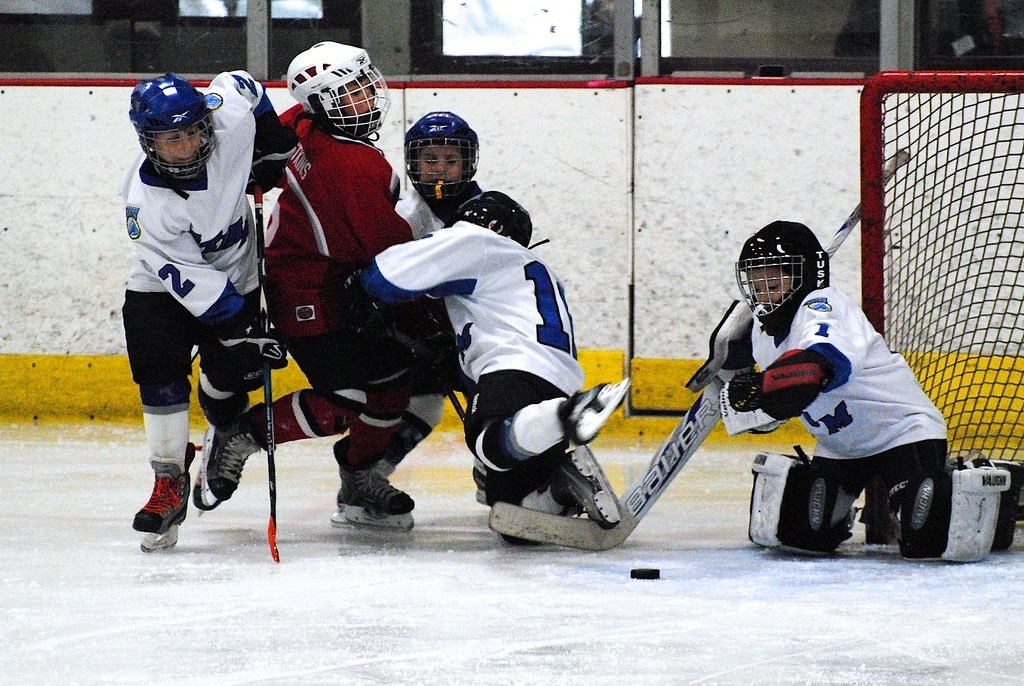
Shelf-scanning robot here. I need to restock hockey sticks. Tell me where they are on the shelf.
[487,149,912,551]
[253,184,281,563]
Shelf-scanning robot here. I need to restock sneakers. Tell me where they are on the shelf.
[558,375,631,444]
[192,428,210,516]
[549,445,622,530]
[332,435,415,531]
[132,442,196,554]
[202,403,268,507]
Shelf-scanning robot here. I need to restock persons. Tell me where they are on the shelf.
[121,71,300,554]
[199,41,456,531]
[336,192,634,531]
[92,0,180,72]
[718,221,1021,560]
[334,111,486,478]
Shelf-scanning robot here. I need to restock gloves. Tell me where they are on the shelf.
[340,267,380,315]
[246,125,300,196]
[219,316,287,370]
[391,298,458,356]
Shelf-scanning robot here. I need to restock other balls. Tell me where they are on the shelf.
[631,568,660,580]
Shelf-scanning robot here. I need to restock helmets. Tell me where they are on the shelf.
[458,191,532,249]
[404,112,480,197]
[734,222,829,336]
[129,73,215,180]
[287,40,392,141]
